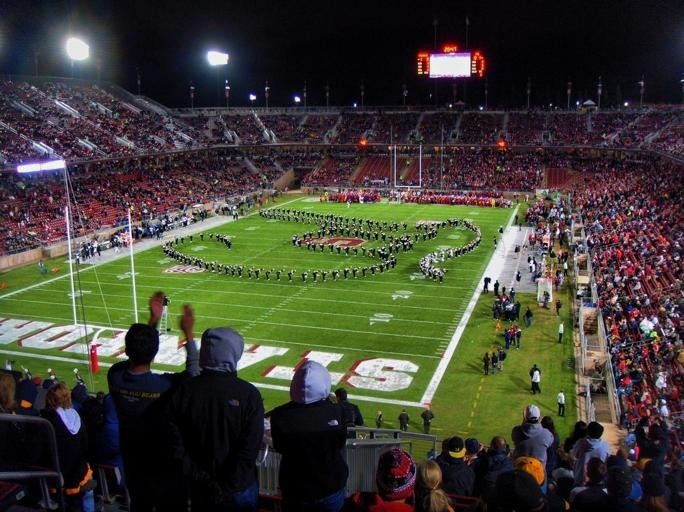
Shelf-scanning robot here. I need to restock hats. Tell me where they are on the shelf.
[524,404,540,420]
[377,448,416,501]
[641,475,669,497]
[589,422,604,439]
[487,472,548,512]
[448,436,465,458]
[465,438,479,454]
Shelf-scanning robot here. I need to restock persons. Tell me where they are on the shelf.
[633,415,670,471]
[5,359,16,371]
[601,446,630,488]
[569,98,684,431]
[589,472,640,512]
[334,387,364,441]
[569,421,610,486]
[162,295,171,313]
[414,459,455,512]
[663,467,684,512]
[22,372,33,384]
[21,364,30,379]
[96,391,106,404]
[430,437,452,462]
[40,380,96,512]
[270,360,349,512]
[436,436,487,498]
[487,469,570,512]
[664,432,684,472]
[340,447,417,512]
[90,340,103,373]
[73,368,88,390]
[511,403,555,468]
[163,326,266,512]
[33,379,55,417]
[563,421,587,453]
[70,379,88,413]
[627,457,653,502]
[0,372,42,512]
[107,290,201,512]
[541,415,563,482]
[624,432,640,461]
[375,409,384,429]
[421,406,434,435]
[464,438,488,466]
[568,456,608,512]
[397,409,410,432]
[637,459,671,512]
[470,435,514,493]
[14,379,40,417]
[556,388,567,418]
[479,100,568,396]
[47,367,60,385]
[32,376,42,393]
[551,449,579,483]
